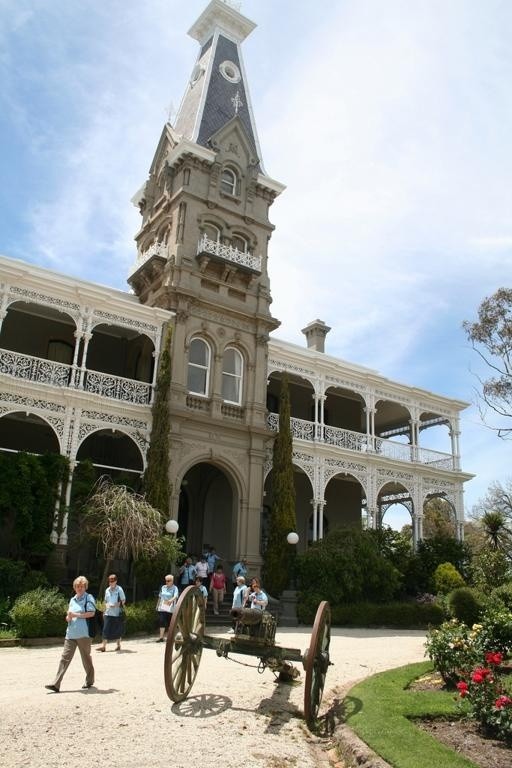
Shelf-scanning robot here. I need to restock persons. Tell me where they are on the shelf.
[45,576,97,692]
[95,574,126,652]
[178,546,280,640]
[153,574,179,644]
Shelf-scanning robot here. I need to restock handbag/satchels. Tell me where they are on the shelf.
[84,592,104,639]
[119,600,128,626]
[188,565,196,584]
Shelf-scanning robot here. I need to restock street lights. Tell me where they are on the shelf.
[164,518,181,574]
[285,531,303,589]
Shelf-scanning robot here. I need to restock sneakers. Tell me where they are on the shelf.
[156,637,164,643]
[45,685,60,692]
[82,682,93,688]
[94,646,105,652]
[114,646,120,651]
